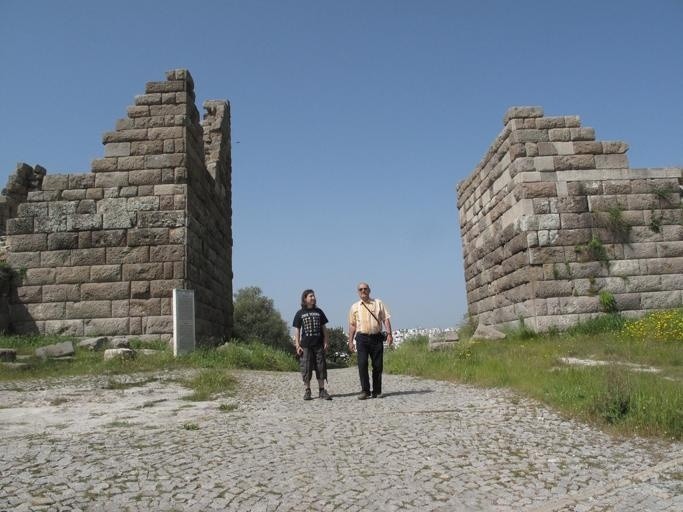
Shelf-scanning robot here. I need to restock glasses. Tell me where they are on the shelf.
[358,288,369,290]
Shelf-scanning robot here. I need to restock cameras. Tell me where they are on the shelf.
[382,330,388,342]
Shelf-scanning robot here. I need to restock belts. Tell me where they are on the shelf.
[360,334,381,337]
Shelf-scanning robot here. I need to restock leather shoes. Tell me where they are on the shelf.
[357,390,371,400]
[372,391,382,398]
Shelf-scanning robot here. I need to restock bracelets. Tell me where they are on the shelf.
[296,346,299,349]
[386,332,391,337]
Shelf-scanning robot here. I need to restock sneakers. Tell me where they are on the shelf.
[319,389,331,400]
[303,390,311,400]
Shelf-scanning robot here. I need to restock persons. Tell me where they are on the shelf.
[346,282,393,400]
[291,289,333,402]
